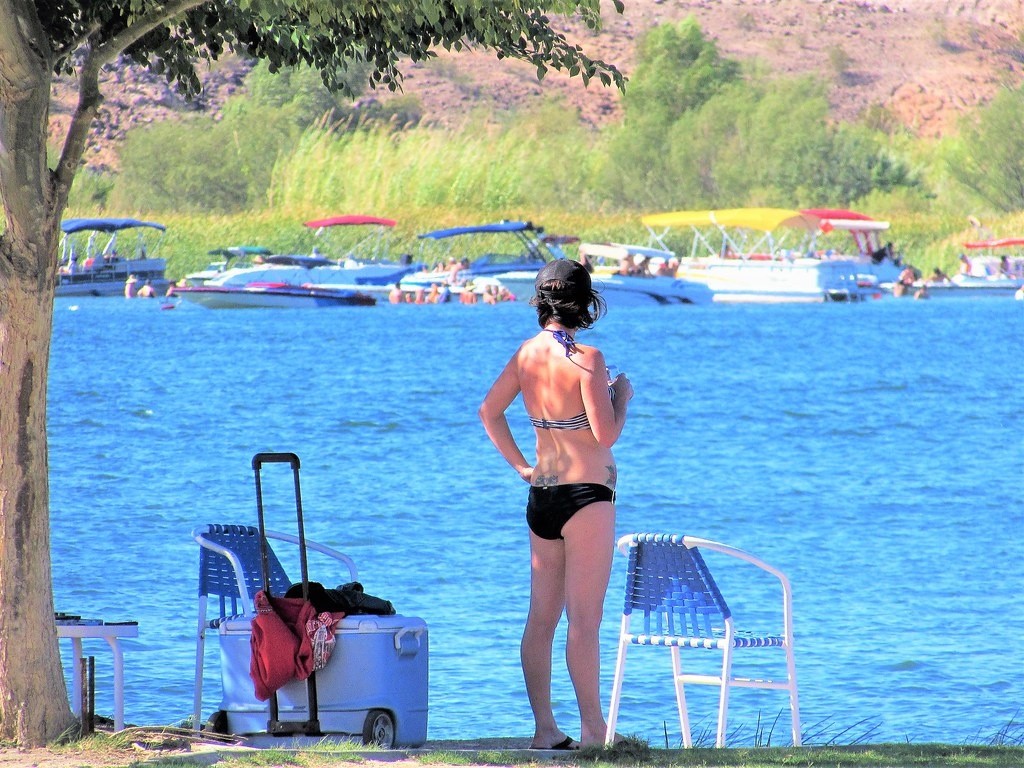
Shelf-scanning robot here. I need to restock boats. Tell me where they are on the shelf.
[880,236,1023,296]
[51,218,171,300]
[172,210,916,311]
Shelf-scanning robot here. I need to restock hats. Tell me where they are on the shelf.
[535,258,592,294]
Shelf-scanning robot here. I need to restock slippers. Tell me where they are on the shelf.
[532,732,582,753]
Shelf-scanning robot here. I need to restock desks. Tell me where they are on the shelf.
[54,611,139,734]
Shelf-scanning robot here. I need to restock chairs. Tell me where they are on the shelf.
[191,523,358,741]
[604,533,802,749]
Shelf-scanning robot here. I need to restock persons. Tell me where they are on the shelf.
[309,249,322,259]
[959,253,972,275]
[253,255,263,267]
[478,258,642,752]
[870,247,888,264]
[389,280,450,304]
[656,259,681,279]
[926,267,954,283]
[124,275,187,298]
[1000,255,1015,279]
[423,256,472,287]
[894,266,914,297]
[578,249,653,277]
[888,242,903,267]
[460,284,476,305]
[914,285,929,299]
[483,284,515,306]
[59,250,117,275]
[718,241,738,258]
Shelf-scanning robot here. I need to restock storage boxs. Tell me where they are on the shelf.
[218,615,429,749]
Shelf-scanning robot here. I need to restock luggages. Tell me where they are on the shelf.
[205,452,431,750]
